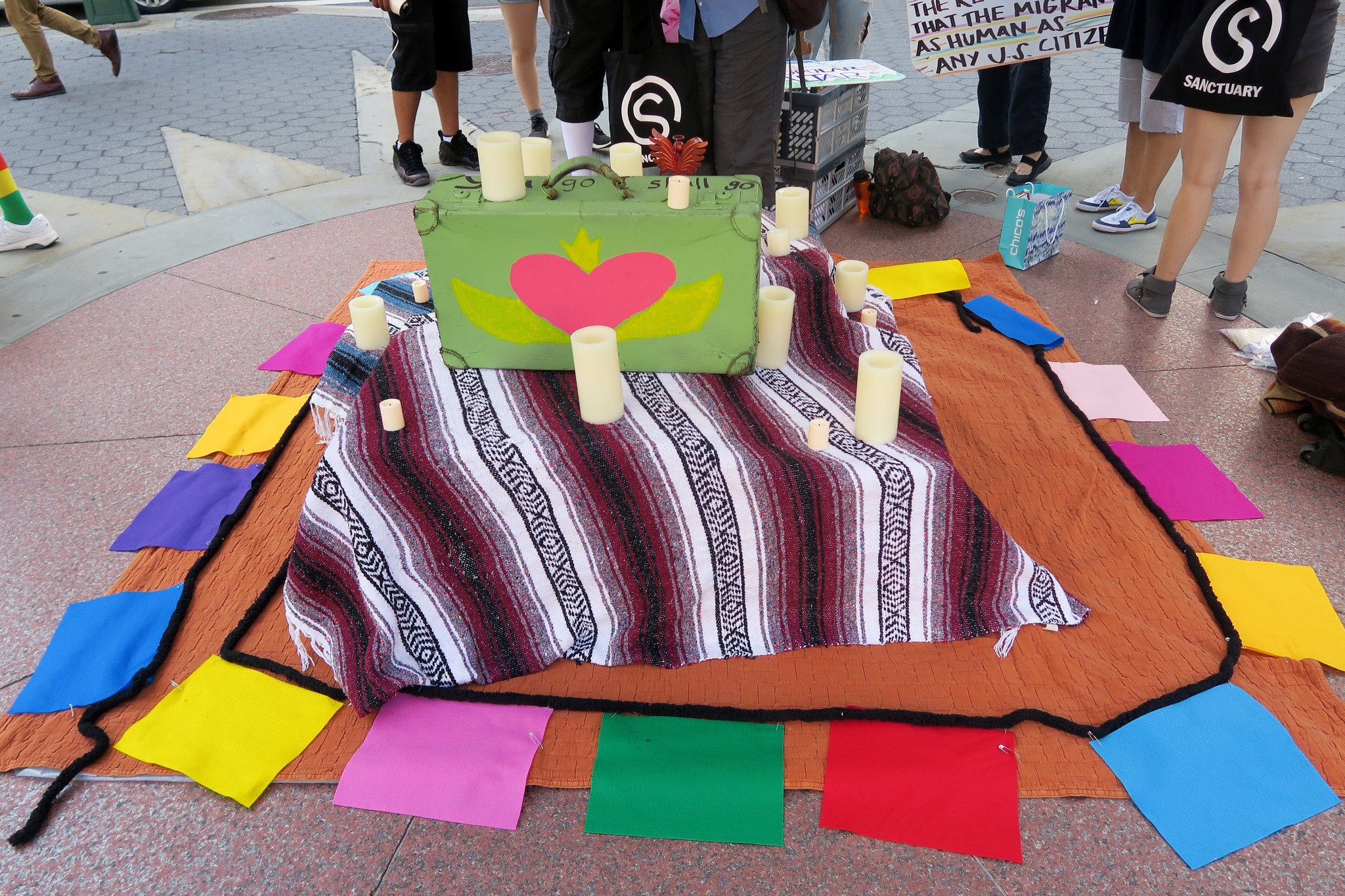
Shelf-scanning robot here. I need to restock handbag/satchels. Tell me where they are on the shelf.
[869,148,952,229]
[998,182,1073,271]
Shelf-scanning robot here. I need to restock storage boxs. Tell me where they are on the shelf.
[774,137,866,235]
[774,83,870,171]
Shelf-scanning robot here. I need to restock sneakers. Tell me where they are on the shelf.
[1092,201,1159,234]
[592,121,611,149]
[0,214,59,252]
[437,129,480,170]
[392,136,430,186]
[1075,184,1135,212]
[529,117,550,138]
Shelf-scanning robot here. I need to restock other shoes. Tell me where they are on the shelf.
[959,147,1013,164]
[8,76,66,99]
[98,28,122,78]
[1209,270,1252,320]
[1005,149,1052,187]
[1125,265,1177,317]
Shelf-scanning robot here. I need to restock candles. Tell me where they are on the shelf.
[570,325,624,424]
[348,295,391,351]
[767,228,790,257]
[861,308,877,328]
[476,131,526,201]
[756,285,795,370]
[412,280,430,303]
[379,399,405,432]
[668,175,690,210]
[807,418,830,450]
[834,260,869,313]
[609,141,643,176]
[776,186,809,241]
[854,349,903,444]
[520,137,552,176]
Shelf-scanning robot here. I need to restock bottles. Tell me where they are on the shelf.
[853,169,874,214]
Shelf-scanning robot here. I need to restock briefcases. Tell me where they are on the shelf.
[414,155,763,376]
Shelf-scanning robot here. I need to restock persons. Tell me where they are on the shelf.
[1,0,122,251]
[368,0,478,187]
[499,0,1339,322]
